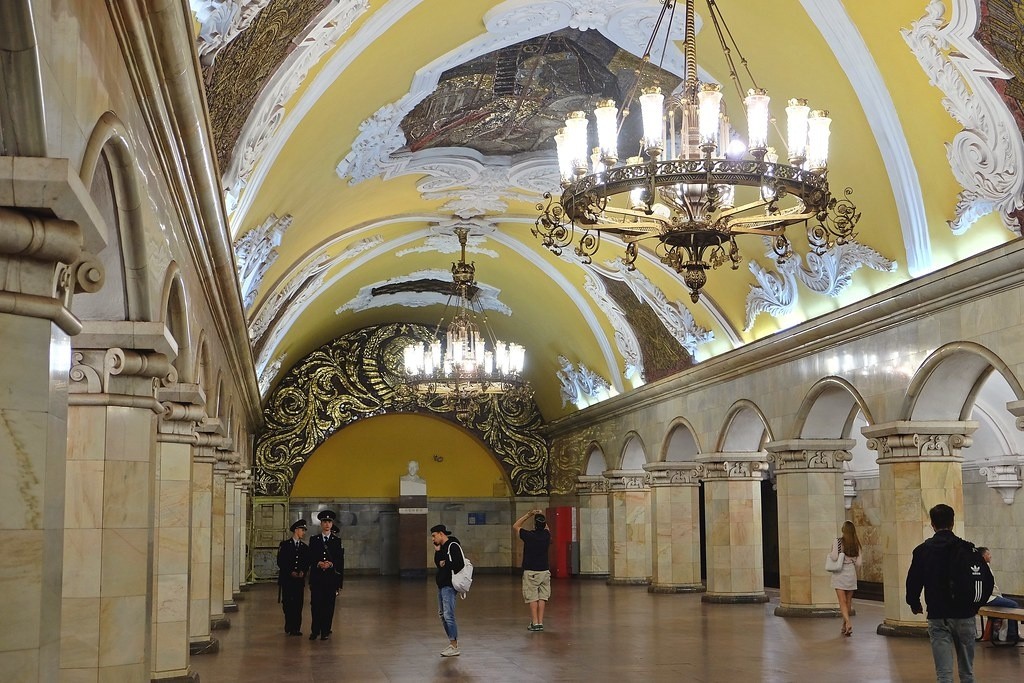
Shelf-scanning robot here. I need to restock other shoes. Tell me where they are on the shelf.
[290,630,303,636]
[1006,634,1024,642]
[321,634,328,640]
[309,633,320,640]
[841,627,846,633]
[845,627,853,636]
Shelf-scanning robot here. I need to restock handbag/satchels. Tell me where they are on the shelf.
[825,538,845,572]
[447,542,473,600]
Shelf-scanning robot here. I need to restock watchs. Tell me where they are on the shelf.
[442,562,445,566]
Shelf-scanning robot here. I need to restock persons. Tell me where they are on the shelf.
[277,520,309,636]
[906,504,994,683]
[330,524,344,596]
[308,510,341,640]
[513,509,551,631]
[825,520,862,637]
[430,525,473,657]
[400,459,427,495]
[977,547,1024,642]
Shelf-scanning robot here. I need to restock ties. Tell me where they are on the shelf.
[296,542,299,550]
[324,536,328,542]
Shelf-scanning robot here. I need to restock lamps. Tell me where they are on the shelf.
[401,226,527,399]
[434,454,444,463]
[530,0,862,304]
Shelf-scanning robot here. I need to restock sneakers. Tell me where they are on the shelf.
[538,624,543,631]
[527,622,539,631]
[441,644,460,657]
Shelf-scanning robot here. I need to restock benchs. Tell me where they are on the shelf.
[977,607,1024,641]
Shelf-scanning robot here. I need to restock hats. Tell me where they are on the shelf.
[430,525,452,535]
[330,524,340,533]
[317,510,336,521]
[291,519,307,531]
